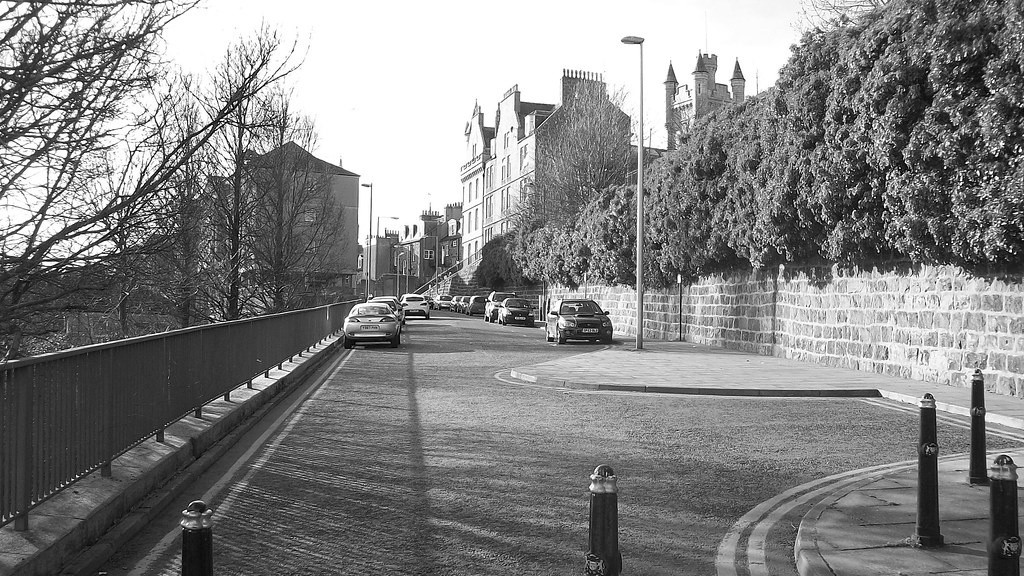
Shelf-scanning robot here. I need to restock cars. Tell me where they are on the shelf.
[450,296,463,313]
[498,298,536,327]
[344,303,401,349]
[372,296,408,325]
[401,294,430,320]
[424,296,434,309]
[366,300,402,333]
[433,295,453,310]
[545,298,613,345]
[457,296,472,314]
[466,295,488,316]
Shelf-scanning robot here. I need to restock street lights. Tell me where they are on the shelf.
[621,36,644,348]
[376,217,399,279]
[361,183,373,299]
[422,236,438,295]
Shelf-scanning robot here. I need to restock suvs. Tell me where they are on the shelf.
[484,291,519,323]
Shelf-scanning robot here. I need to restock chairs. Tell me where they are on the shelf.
[562,306,590,312]
